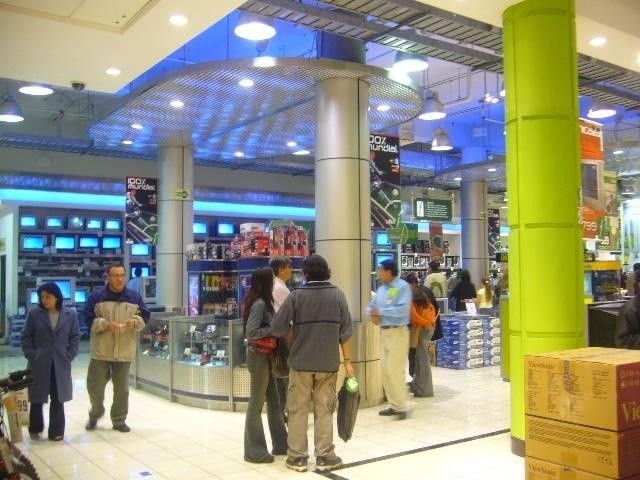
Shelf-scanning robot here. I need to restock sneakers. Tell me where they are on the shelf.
[316,456,343,472]
[48,435,64,441]
[113,424,129,432]
[86,406,104,429]
[271,445,289,454]
[379,407,407,420]
[29,431,40,440]
[246,452,274,463]
[284,457,308,472]
[407,381,432,397]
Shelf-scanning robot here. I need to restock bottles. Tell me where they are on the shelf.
[623,272,627,282]
[620,288,625,299]
[240,338,248,368]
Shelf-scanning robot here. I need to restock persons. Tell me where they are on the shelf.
[82,262,150,432]
[241,266,289,464]
[403,259,501,397]
[267,255,294,424]
[364,258,411,422]
[19,283,80,441]
[613,267,640,350]
[268,254,354,472]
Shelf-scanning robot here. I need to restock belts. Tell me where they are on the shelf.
[381,326,398,329]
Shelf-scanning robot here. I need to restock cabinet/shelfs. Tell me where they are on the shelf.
[401,252,461,284]
[128,319,249,412]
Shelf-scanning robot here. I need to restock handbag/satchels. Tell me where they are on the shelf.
[409,326,419,348]
[247,337,276,354]
[271,339,291,378]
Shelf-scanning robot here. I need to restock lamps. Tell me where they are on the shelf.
[392,49,433,74]
[429,124,457,153]
[585,97,618,122]
[234,11,276,41]
[416,73,451,124]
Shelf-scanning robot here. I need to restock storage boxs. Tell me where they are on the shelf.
[523,458,639,480]
[523,345,639,480]
[428,296,500,370]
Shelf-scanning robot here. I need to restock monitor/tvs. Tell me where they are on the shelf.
[87,219,104,229]
[26,288,40,305]
[102,237,121,251]
[37,277,76,305]
[56,235,75,251]
[193,221,210,235]
[214,220,236,235]
[78,290,86,302]
[46,214,65,229]
[107,217,120,230]
[377,254,394,264]
[375,230,388,246]
[130,267,149,276]
[80,234,100,251]
[68,213,84,230]
[130,243,150,256]
[21,212,38,229]
[20,233,46,251]
